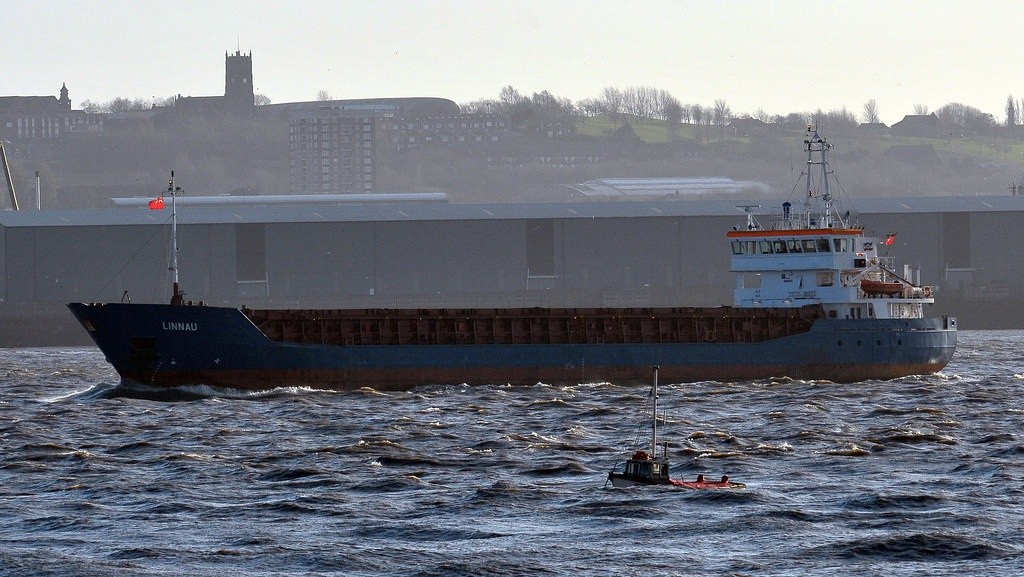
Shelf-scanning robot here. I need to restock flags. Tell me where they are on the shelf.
[885,233,897,246]
[149,195,165,210]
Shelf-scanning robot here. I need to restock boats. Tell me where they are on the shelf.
[65,127,960,391]
[609,365,747,490]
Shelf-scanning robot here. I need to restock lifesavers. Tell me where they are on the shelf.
[924,288,931,297]
